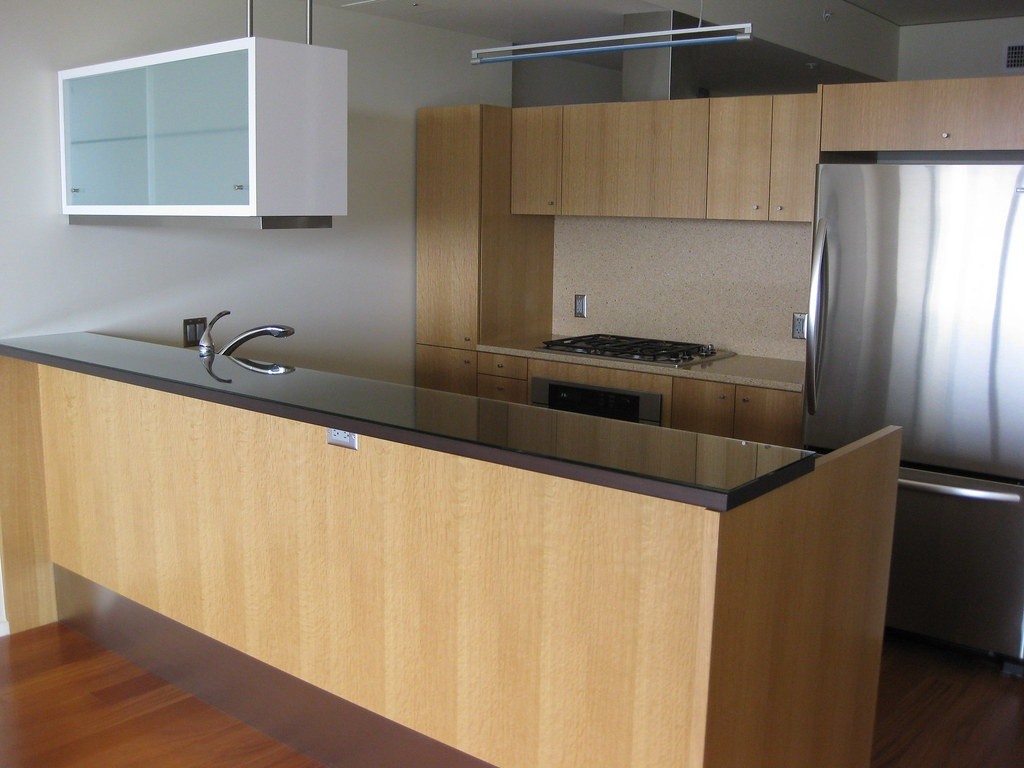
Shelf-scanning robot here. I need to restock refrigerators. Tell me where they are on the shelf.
[797,147,1024,768]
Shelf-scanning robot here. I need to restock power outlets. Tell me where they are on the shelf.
[328,427,357,449]
[575,295,586,318]
[792,313,808,339]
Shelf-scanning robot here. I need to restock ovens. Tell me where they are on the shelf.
[529,377,664,427]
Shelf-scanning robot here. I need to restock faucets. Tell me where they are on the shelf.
[197,353,295,384]
[198,311,295,352]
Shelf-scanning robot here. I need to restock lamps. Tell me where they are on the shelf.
[470,0,752,65]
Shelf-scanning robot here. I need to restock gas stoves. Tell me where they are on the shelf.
[542,333,717,363]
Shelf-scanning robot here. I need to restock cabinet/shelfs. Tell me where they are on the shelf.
[511,92,818,222]
[671,376,804,449]
[59,37,347,229]
[416,343,527,404]
[411,101,554,351]
